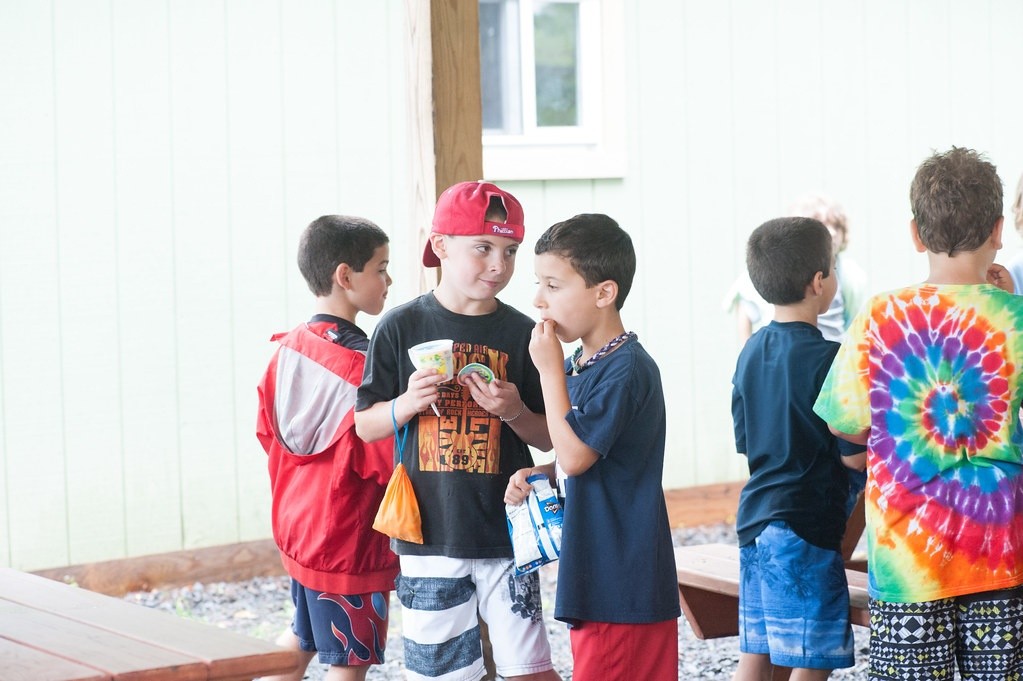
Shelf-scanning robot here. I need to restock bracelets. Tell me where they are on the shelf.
[500,402,526,422]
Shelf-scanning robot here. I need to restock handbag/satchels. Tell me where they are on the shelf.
[372,397,424,545]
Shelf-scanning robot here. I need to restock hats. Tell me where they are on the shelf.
[421,179,526,269]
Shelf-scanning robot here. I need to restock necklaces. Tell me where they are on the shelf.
[571,331,635,374]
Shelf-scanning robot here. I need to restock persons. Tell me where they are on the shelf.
[354,179,561,681]
[732,216,868,681]
[796,201,860,344]
[813,149,1023,679]
[986,164,1023,295]
[255,215,404,681]
[505,213,681,681]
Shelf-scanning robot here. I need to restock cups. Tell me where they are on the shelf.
[405,339,456,385]
